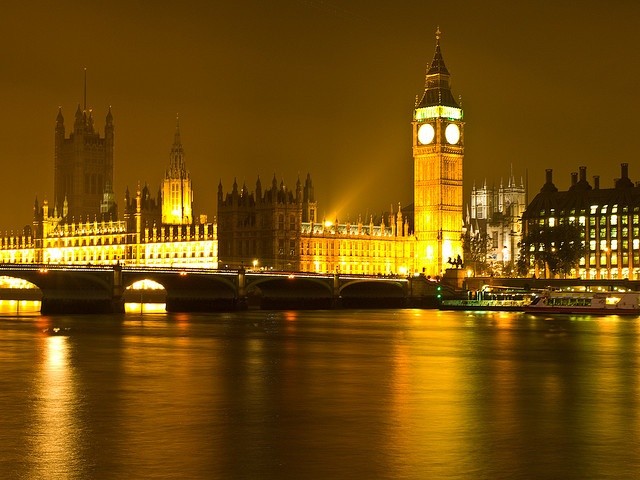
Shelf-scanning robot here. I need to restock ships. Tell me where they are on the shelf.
[524,292,640,314]
[440,292,534,311]
